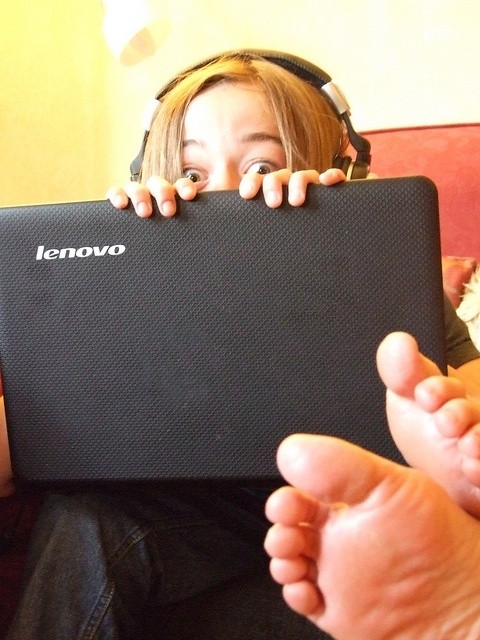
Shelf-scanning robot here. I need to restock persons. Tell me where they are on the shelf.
[258,329,480,633]
[3,46,480,633]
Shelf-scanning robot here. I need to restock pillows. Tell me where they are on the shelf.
[440,256,476,309]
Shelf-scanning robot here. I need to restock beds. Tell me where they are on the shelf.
[347,122,480,354]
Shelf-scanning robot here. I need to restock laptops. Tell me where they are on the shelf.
[0,175,448,490]
